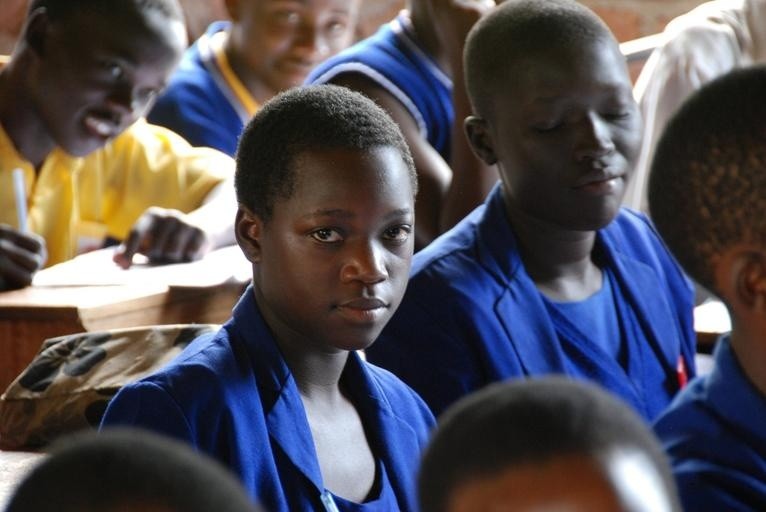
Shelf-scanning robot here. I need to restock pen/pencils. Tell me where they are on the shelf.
[13,167,28,236]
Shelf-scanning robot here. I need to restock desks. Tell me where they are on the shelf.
[0,251,246,389]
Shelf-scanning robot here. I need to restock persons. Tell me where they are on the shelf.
[644,62,766,512]
[3,425,265,512]
[0,0,239,294]
[362,0,694,431]
[302,0,509,251]
[619,0,765,304]
[416,375,687,512]
[147,0,360,157]
[92,82,440,511]
